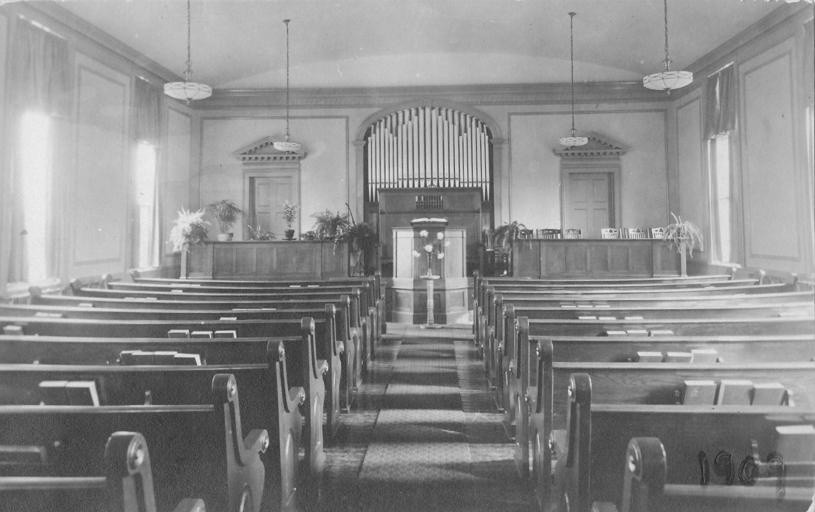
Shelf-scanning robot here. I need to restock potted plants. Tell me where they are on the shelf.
[663,212,705,276]
[313,210,348,239]
[171,206,211,281]
[211,200,241,242]
[281,201,295,238]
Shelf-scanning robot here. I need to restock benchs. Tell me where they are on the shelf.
[3,273,381,511]
[472,268,809,508]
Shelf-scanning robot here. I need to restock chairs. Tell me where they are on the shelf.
[518,226,669,242]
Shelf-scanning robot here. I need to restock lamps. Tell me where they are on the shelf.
[412,227,450,275]
[164,1,210,101]
[560,13,587,147]
[641,3,693,96]
[273,22,302,150]
